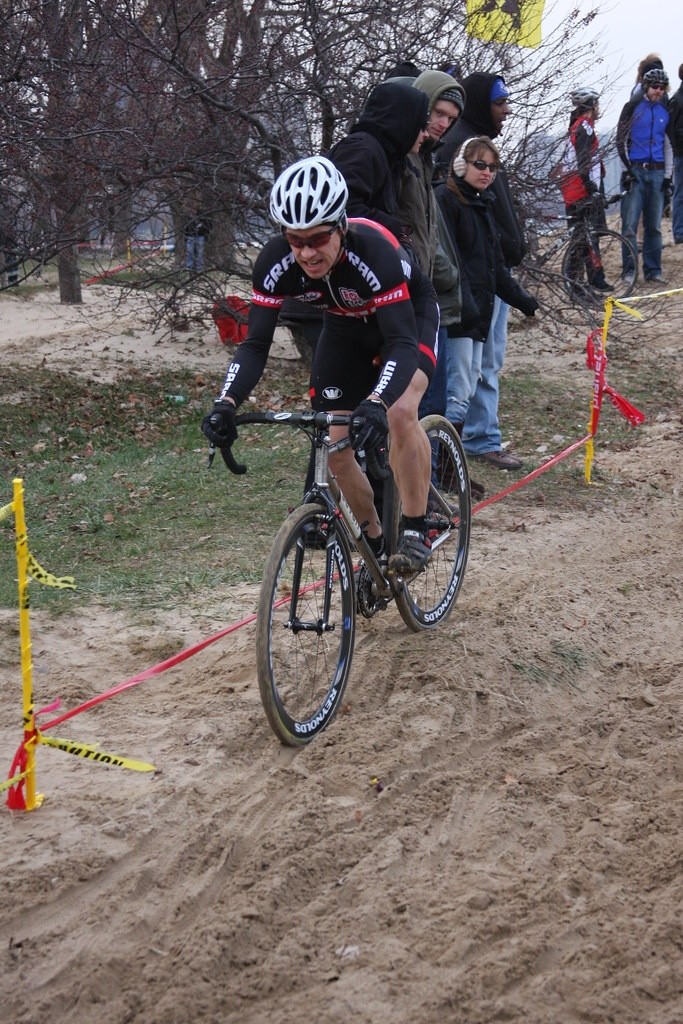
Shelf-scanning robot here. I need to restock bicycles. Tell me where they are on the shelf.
[514,190,638,315]
[205,352,473,743]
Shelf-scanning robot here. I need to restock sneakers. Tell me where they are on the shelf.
[402,523,431,571]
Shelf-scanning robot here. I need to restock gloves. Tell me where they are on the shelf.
[592,190,609,211]
[660,178,671,191]
[200,399,239,449]
[622,172,640,192]
[348,399,390,458]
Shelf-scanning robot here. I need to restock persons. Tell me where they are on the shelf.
[279,61,540,520]
[560,85,616,300]
[202,156,442,585]
[0,188,20,287]
[183,201,210,283]
[616,55,683,287]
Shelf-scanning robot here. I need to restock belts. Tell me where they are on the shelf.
[631,162,664,169]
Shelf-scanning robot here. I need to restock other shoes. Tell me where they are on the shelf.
[588,280,614,291]
[644,275,669,285]
[624,277,639,290]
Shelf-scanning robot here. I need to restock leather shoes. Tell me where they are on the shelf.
[471,451,522,469]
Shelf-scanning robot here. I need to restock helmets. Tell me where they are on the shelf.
[571,86,599,106]
[643,69,669,85]
[269,156,349,230]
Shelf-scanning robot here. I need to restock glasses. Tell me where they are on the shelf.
[649,84,665,90]
[282,213,344,248]
[466,159,498,172]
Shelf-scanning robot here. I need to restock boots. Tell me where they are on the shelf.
[437,420,484,500]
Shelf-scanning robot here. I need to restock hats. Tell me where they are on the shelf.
[491,78,509,103]
[439,88,464,112]
